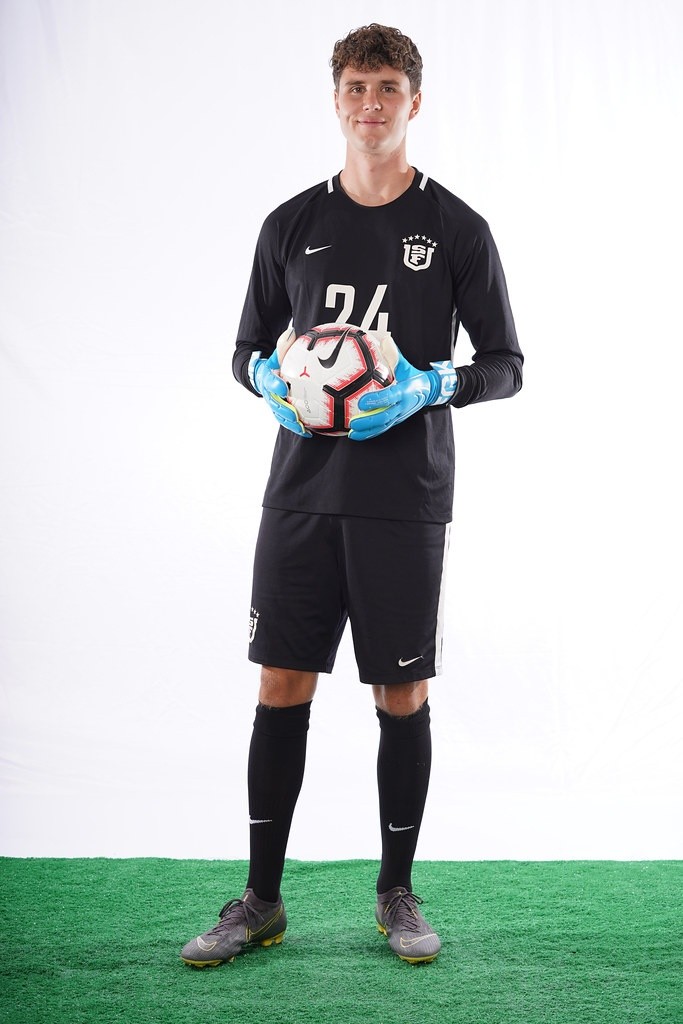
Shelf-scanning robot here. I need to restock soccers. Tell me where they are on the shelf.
[280,323,394,437]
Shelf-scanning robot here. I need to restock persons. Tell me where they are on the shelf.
[176,19,531,958]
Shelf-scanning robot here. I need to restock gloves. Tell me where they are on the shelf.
[246,327,313,438]
[347,338,457,440]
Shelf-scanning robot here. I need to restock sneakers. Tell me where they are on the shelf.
[375,886,441,964]
[180,888,287,966]
